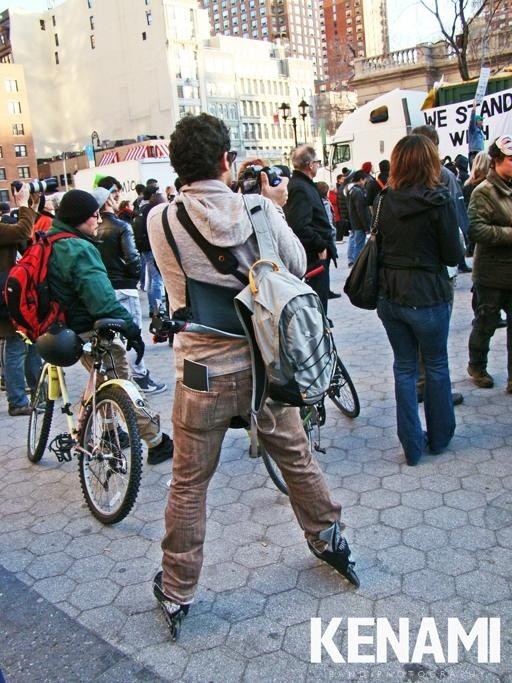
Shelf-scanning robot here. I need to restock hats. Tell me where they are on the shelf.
[143,186,160,199]
[90,185,114,209]
[56,189,100,227]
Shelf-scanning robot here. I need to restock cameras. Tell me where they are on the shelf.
[240,165,290,194]
[11,178,58,196]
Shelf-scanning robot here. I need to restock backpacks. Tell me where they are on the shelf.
[232,192,338,415]
[3,229,82,343]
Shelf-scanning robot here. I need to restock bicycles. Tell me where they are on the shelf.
[260,258,359,498]
[23,315,146,528]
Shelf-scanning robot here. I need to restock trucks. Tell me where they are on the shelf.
[318,72,512,190]
[39,158,179,223]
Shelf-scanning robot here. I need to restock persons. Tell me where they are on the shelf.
[467,98,487,171]
[1,127,512,418]
[372,132,466,469]
[44,188,175,466]
[145,110,362,624]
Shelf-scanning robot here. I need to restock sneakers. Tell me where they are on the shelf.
[147,432,174,465]
[451,393,464,406]
[9,402,44,417]
[99,424,131,455]
[132,369,168,396]
[467,359,495,388]
[405,430,427,467]
[505,379,512,395]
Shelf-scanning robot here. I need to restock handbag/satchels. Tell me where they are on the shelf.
[343,233,380,311]
[180,280,249,338]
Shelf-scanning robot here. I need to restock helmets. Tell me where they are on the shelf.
[35,320,84,367]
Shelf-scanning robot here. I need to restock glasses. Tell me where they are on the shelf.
[228,150,237,163]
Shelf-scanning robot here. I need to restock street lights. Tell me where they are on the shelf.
[278,99,308,151]
[283,151,291,171]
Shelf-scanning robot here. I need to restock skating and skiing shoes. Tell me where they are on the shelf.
[306,520,361,589]
[152,570,190,642]
